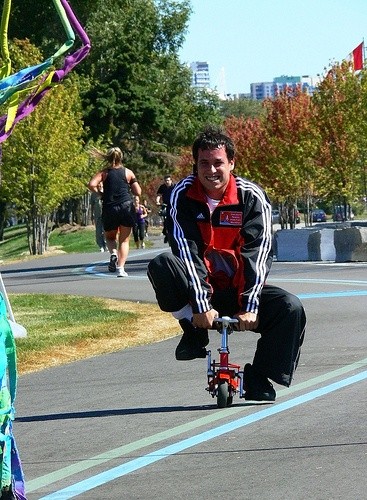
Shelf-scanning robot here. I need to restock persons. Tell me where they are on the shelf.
[90,147,142,277]
[89,182,108,252]
[146,131,306,401]
[155,176,176,244]
[132,195,151,249]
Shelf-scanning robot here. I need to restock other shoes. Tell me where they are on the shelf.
[116,267,128,277]
[175,318,210,361]
[243,362,276,401]
[108,253,118,272]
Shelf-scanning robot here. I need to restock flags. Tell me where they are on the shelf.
[351,43,363,72]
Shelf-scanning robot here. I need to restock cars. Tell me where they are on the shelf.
[309,209,327,223]
[272,209,280,224]
[330,205,355,222]
[288,212,301,224]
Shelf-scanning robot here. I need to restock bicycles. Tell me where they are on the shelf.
[190,315,259,409]
